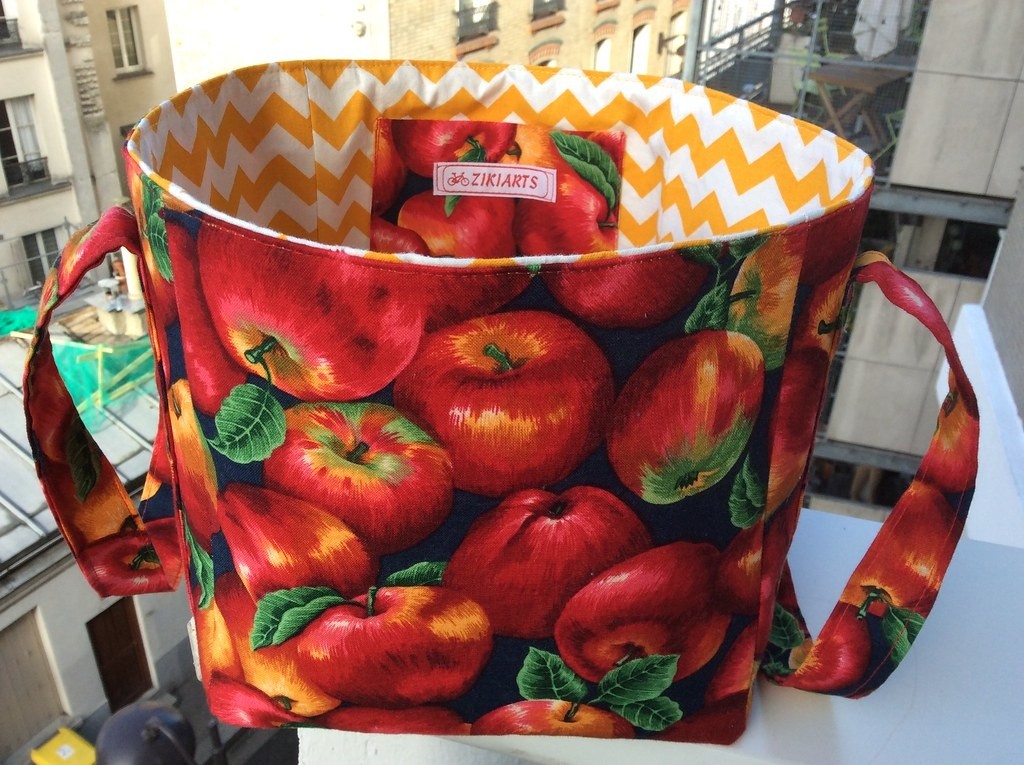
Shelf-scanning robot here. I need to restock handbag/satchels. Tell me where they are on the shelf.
[20,60,980,745]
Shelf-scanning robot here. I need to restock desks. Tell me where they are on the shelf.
[809,56,910,153]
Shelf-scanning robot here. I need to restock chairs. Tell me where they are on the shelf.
[787,16,905,167]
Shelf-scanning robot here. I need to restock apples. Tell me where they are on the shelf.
[26,115,979,744]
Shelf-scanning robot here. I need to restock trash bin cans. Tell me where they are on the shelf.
[30,726,95,765]
